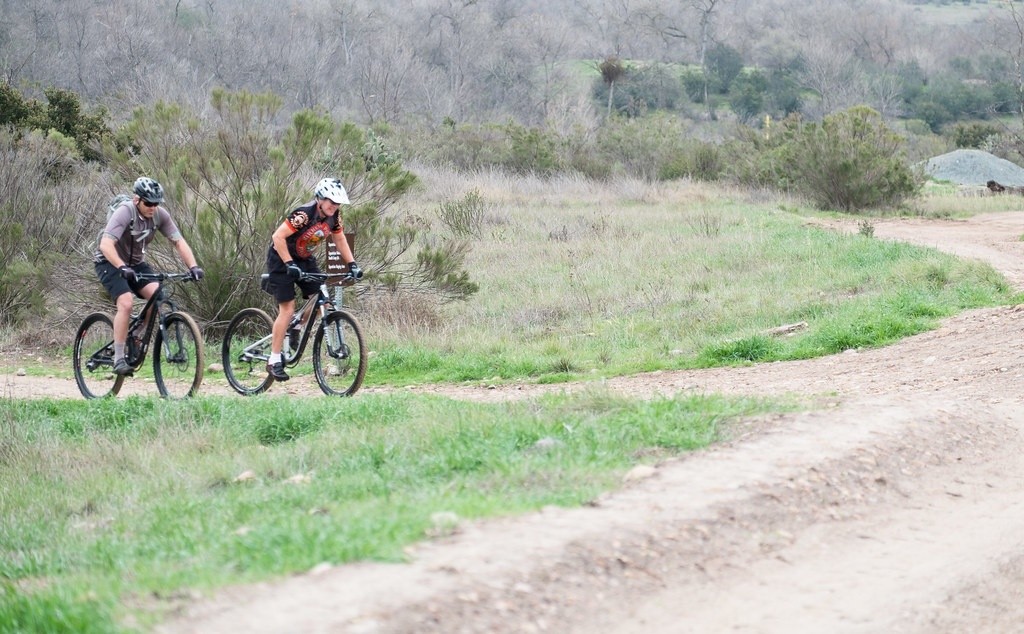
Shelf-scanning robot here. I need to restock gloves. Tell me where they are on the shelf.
[284,261,308,282]
[118,264,139,284]
[346,262,363,282]
[191,265,205,281]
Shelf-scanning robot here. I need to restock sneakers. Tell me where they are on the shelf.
[113,359,135,377]
[133,336,141,356]
[288,328,301,352]
[266,358,289,381]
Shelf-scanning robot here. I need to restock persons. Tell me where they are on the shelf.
[93,176,205,375]
[265,178,363,382]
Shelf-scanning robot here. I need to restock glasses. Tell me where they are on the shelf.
[139,198,159,207]
[329,199,340,206]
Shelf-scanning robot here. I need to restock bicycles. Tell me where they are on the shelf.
[221,269,368,399]
[73,272,203,401]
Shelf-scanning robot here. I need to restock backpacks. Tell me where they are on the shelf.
[105,195,162,243]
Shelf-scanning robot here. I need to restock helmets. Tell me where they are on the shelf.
[133,177,166,203]
[314,178,350,205]
